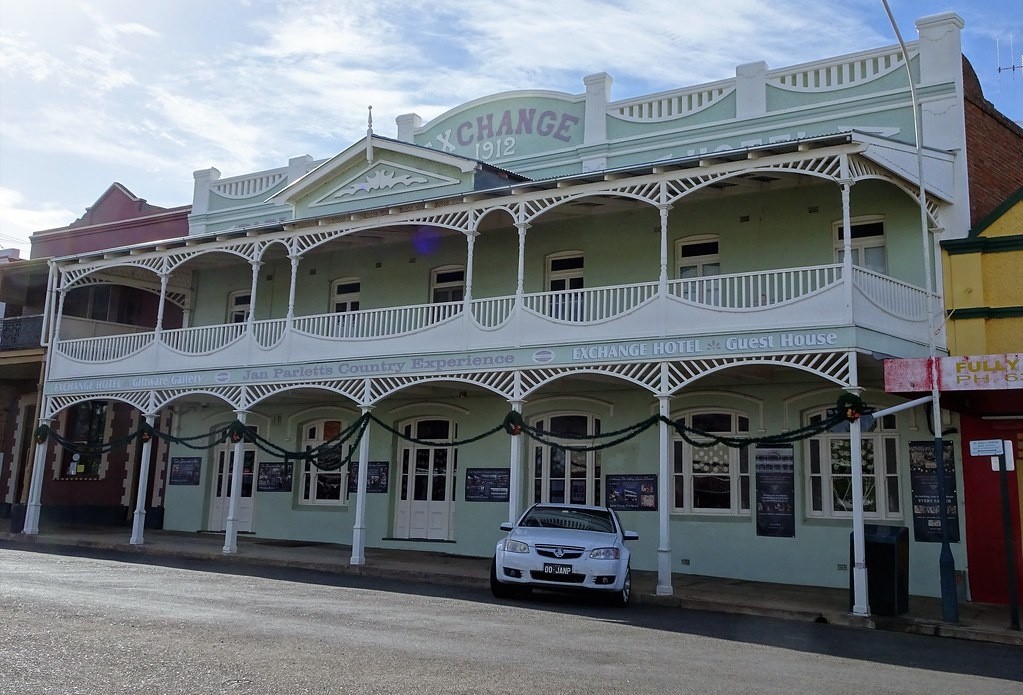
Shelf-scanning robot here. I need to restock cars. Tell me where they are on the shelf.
[490,502,640,606]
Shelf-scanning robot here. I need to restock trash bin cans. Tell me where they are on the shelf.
[850,524,909,617]
